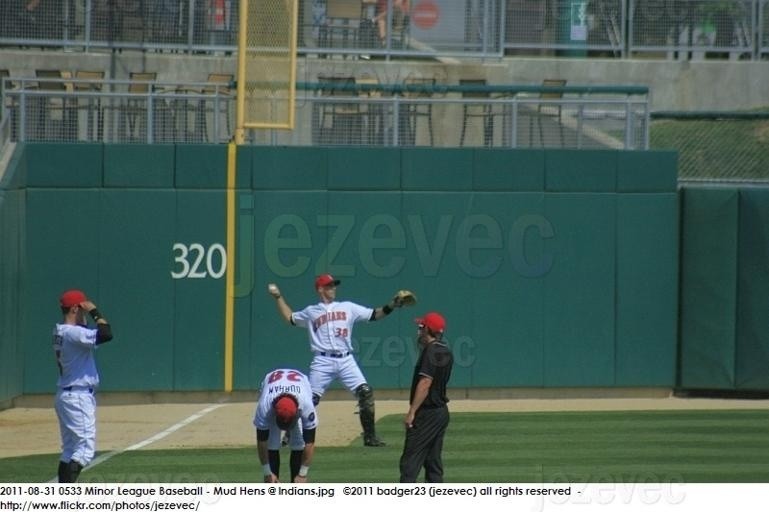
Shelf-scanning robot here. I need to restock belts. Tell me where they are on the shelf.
[319,350,352,359]
[62,386,93,393]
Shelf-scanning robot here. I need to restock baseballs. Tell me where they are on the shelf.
[269,285,276,290]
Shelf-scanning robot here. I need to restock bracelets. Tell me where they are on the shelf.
[276,294,282,299]
[383,305,393,315]
[89,308,103,321]
[298,465,310,477]
[262,463,272,475]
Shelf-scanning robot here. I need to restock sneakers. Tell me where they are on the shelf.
[364,434,386,448]
[273,395,299,432]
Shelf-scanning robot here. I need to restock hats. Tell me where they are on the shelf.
[313,273,341,288]
[413,311,447,333]
[59,288,93,308]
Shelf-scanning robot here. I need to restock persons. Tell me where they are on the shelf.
[399,311,453,483]
[49,289,113,483]
[267,272,417,448]
[2,2,409,60]
[253,368,319,482]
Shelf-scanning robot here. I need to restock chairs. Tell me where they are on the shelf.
[517,80,566,148]
[457,79,513,148]
[0,0,437,149]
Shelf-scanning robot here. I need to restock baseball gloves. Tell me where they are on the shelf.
[394,290,416,306]
